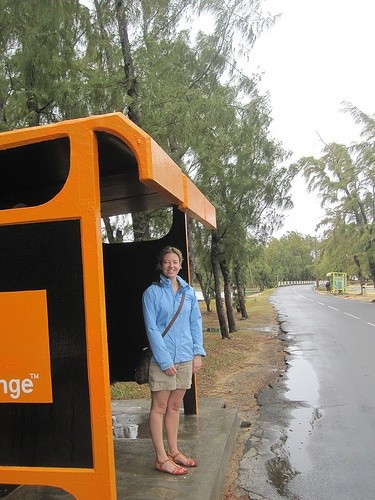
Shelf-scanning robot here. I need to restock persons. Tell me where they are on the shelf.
[325,281,331,293]
[142,246,207,473]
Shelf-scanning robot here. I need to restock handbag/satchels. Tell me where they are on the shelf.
[135,346,153,385]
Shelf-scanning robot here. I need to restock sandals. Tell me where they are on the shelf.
[155,452,198,474]
